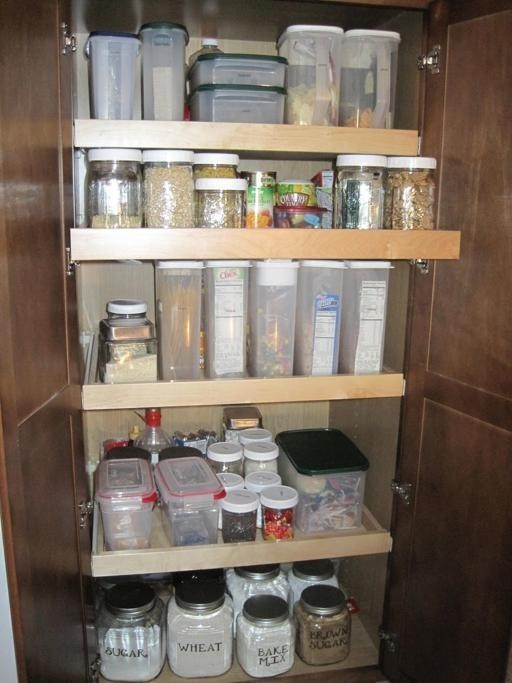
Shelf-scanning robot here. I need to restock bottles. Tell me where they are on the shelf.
[93,559,353,677]
[206,427,297,540]
[188,36,223,69]
[97,297,160,383]
[88,149,436,229]
[136,408,174,506]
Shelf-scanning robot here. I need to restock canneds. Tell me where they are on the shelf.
[241,171,276,229]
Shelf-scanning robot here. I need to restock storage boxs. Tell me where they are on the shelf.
[89,449,159,552]
[344,28,403,129]
[276,24,344,128]
[273,420,374,536]
[151,451,226,550]
[190,51,289,90]
[189,84,288,126]
[154,253,397,381]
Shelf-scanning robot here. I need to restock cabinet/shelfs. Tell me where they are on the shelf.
[68,113,465,682]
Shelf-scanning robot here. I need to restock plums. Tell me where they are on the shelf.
[274,218,290,228]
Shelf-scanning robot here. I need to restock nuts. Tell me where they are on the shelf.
[385,171,434,230]
